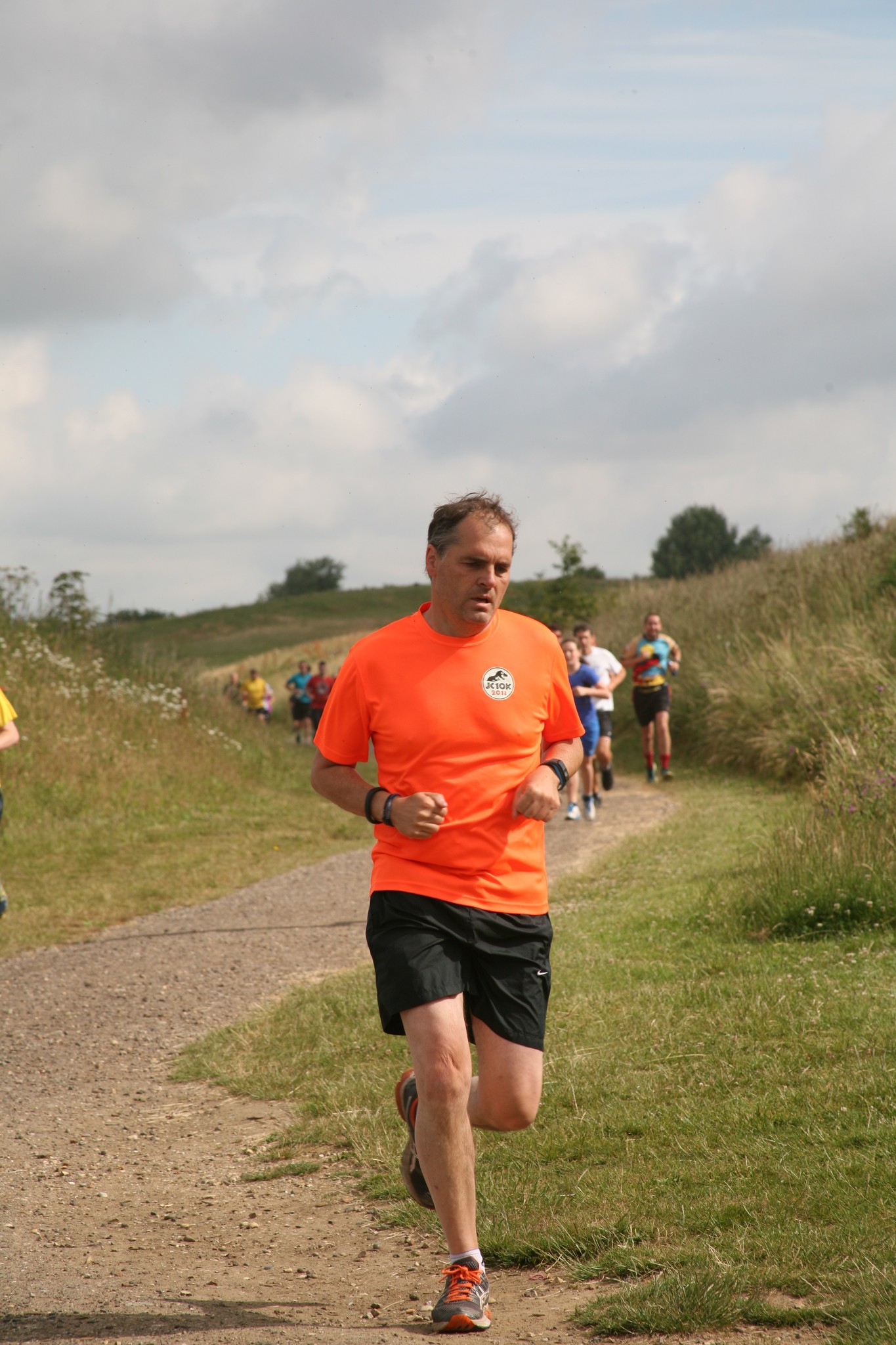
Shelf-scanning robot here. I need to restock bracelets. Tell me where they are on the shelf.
[380,793,401,827]
[364,787,389,824]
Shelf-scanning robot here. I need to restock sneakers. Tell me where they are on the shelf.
[565,803,580,820]
[432,1256,491,1332]
[394,1069,436,1210]
[582,793,596,822]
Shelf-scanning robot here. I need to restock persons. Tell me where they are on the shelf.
[285,661,312,743]
[0,690,20,916]
[550,625,564,645]
[571,625,629,787]
[221,667,273,724]
[310,492,586,1333]
[621,613,682,783]
[560,640,613,820]
[306,662,335,737]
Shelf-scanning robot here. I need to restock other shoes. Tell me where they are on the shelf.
[602,764,613,790]
[592,792,600,806]
[661,770,672,780]
[647,763,658,782]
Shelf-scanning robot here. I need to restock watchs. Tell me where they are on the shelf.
[539,756,570,792]
